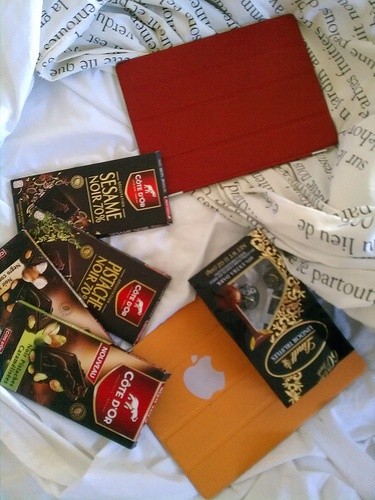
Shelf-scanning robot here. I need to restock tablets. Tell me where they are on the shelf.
[117,15,340,195]
